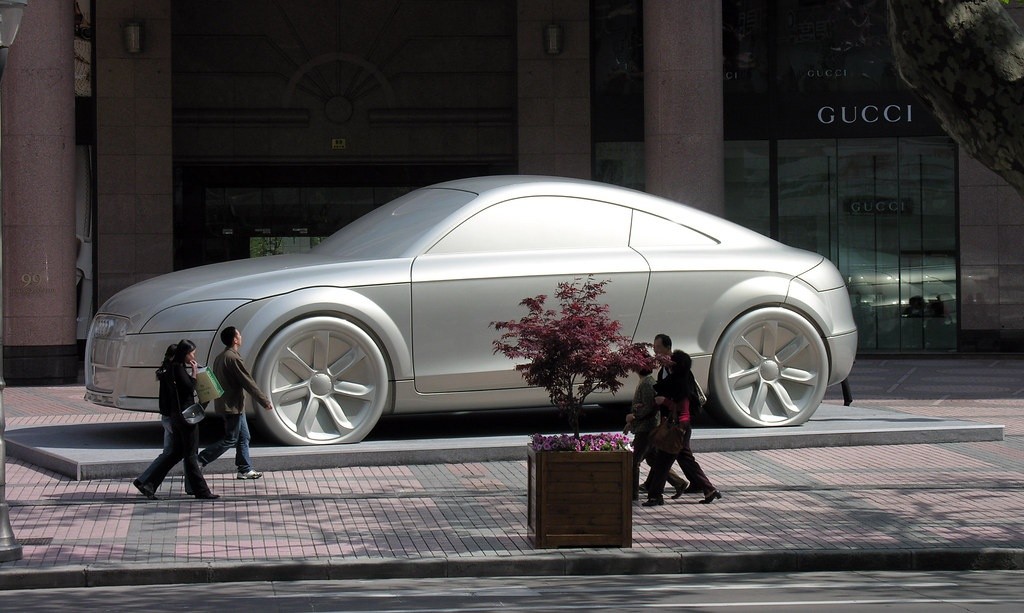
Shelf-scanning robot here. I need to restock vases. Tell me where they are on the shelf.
[527,443,634,550]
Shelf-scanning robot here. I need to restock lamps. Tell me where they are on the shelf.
[123,22,143,53]
[544,24,565,55]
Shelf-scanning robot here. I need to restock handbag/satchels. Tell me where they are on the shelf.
[648,401,686,454]
[192,359,224,404]
[694,379,707,406]
[181,403,207,424]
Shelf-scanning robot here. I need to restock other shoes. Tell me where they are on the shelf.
[639,484,649,493]
[685,484,703,493]
[195,493,220,499]
[133,479,159,500]
[671,482,688,498]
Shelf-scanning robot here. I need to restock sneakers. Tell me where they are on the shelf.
[196,460,203,472]
[237,469,262,479]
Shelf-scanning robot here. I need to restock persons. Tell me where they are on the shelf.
[133,339,221,500]
[623,354,690,500]
[642,350,722,507]
[197,325,273,479]
[640,334,704,494]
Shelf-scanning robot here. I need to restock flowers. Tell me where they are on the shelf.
[488,272,675,452]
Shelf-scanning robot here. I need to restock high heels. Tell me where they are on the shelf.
[699,490,722,504]
[642,495,664,506]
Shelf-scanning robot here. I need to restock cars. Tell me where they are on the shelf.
[79,175,859,444]
